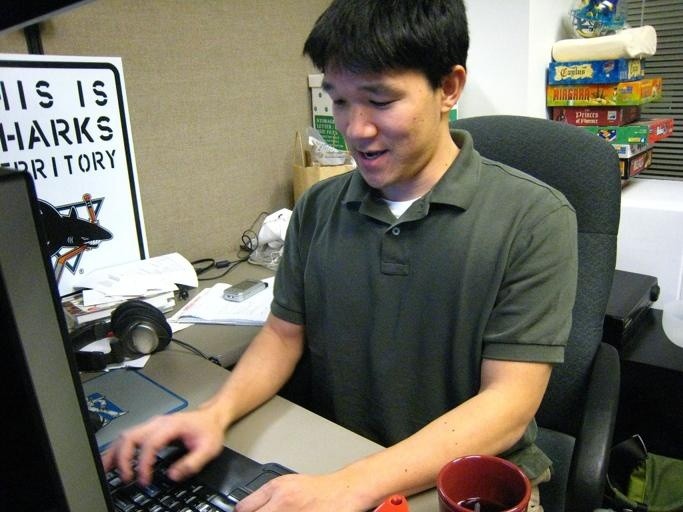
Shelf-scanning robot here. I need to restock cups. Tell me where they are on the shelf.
[437,455,530,510]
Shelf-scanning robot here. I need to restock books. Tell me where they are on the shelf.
[61,251,276,334]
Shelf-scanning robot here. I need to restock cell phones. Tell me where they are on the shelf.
[222,279,268,303]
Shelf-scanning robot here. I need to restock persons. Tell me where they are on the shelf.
[101,0,578,512]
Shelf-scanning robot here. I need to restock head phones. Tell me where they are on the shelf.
[69,300,173,372]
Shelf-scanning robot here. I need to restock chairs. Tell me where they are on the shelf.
[444,115,626,511]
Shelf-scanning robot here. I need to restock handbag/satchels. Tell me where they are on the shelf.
[293,165,354,207]
[623,452,683,512]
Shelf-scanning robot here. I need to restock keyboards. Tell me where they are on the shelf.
[99,435,300,507]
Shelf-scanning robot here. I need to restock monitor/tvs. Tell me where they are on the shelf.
[0,164,113,512]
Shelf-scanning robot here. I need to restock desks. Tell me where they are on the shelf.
[75,239,441,510]
